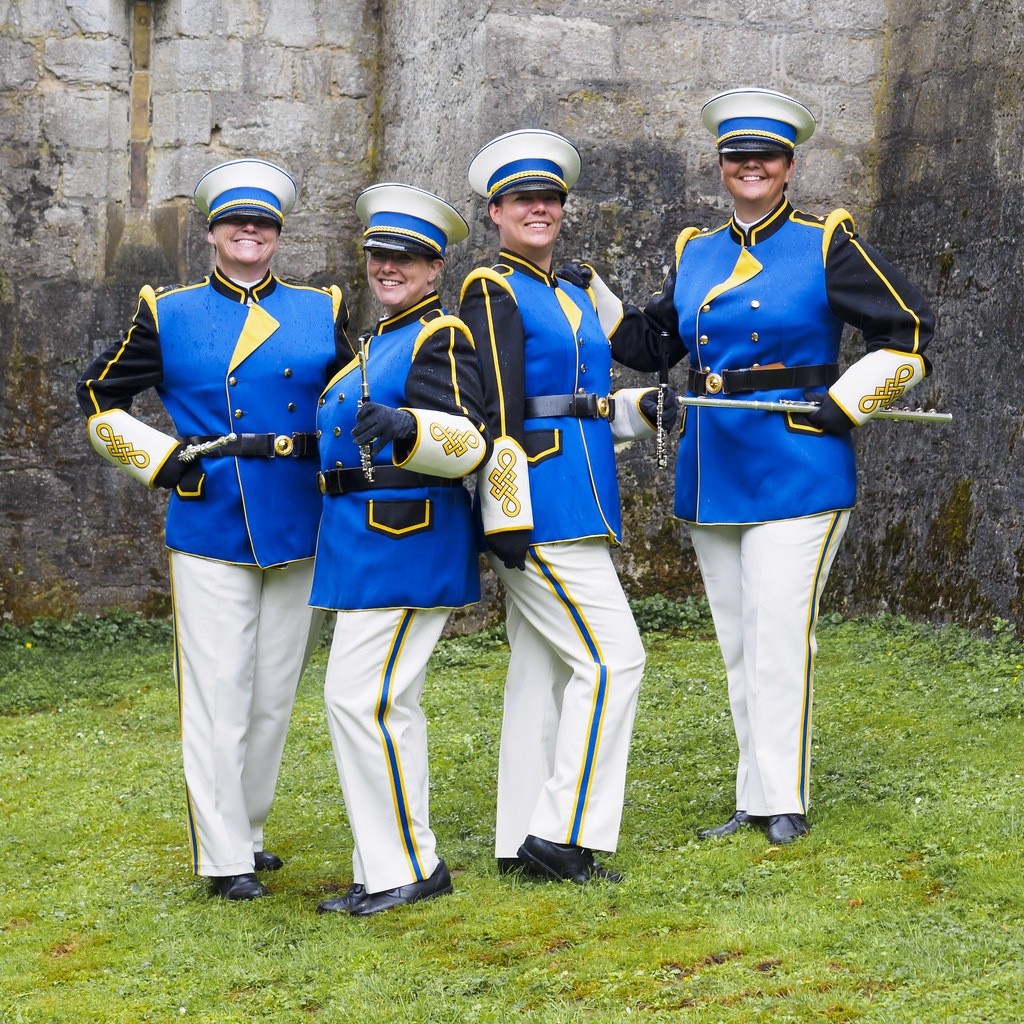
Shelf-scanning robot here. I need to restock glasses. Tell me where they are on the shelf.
[365,248,422,267]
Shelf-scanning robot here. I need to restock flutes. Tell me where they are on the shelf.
[675,395,953,423]
[178,432,238,461]
[656,331,668,471]
[358,337,375,484]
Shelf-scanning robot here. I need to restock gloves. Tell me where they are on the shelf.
[489,529,528,572]
[641,388,679,434]
[556,261,594,288]
[152,444,188,489]
[804,394,855,437]
[350,403,416,457]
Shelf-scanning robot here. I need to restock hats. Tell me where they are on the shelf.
[467,129,583,207]
[354,183,469,261]
[701,88,818,156]
[194,158,296,227]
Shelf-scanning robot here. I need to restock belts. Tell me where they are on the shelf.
[522,393,615,422]
[316,466,466,496]
[687,363,839,395]
[182,433,319,461]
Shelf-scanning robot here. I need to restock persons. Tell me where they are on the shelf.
[76,157,358,902]
[558,87,935,844]
[306,180,494,917]
[460,127,677,890]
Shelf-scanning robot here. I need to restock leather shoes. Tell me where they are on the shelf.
[497,858,527,878]
[759,813,811,843]
[210,874,267,903]
[349,857,453,918]
[316,882,373,915]
[253,851,283,873]
[697,811,767,841]
[516,836,623,888]
